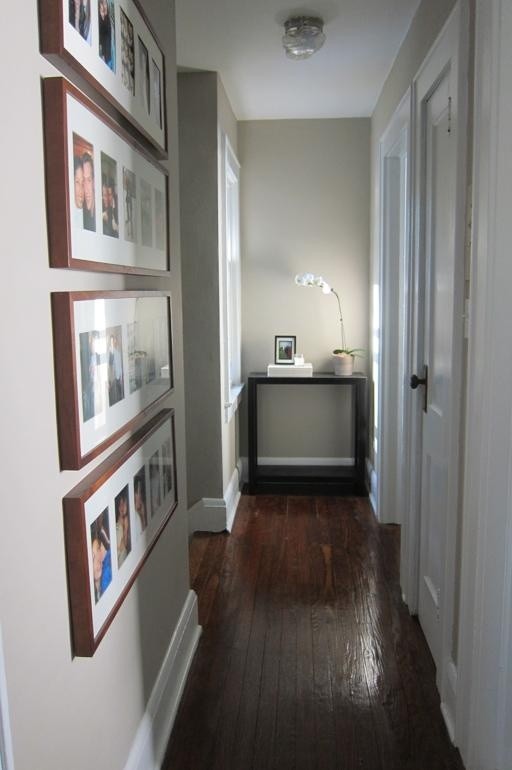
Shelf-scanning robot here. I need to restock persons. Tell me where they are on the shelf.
[69,1,91,41]
[81,333,108,422]
[114,484,132,568]
[147,454,161,517]
[91,509,112,603]
[163,454,170,497]
[120,13,134,91]
[104,336,124,407]
[98,0,111,68]
[133,473,148,536]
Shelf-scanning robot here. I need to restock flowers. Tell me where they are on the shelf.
[295,270,366,350]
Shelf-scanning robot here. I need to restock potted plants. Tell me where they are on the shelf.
[331,349,356,377]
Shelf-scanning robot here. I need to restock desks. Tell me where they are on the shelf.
[247,370,373,494]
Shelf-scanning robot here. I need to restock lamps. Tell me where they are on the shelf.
[282,16,328,63]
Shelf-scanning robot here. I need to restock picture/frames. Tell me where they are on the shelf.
[62,408,180,659]
[37,0,173,161]
[50,289,177,472]
[40,74,173,279]
[275,336,296,364]
